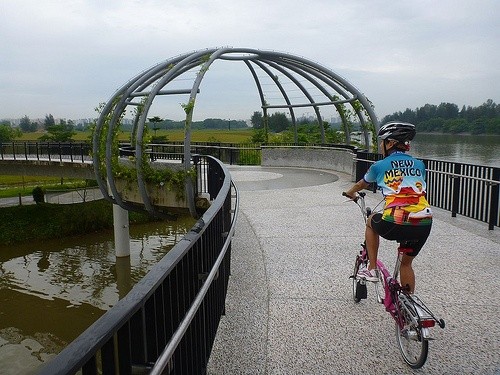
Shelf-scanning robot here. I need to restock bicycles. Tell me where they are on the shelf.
[342,190,445,368]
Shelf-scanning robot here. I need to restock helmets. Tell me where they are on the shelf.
[377,121,416,144]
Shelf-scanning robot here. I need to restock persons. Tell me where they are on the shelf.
[343,121,433,336]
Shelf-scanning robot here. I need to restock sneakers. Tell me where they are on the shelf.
[356,269,379,281]
